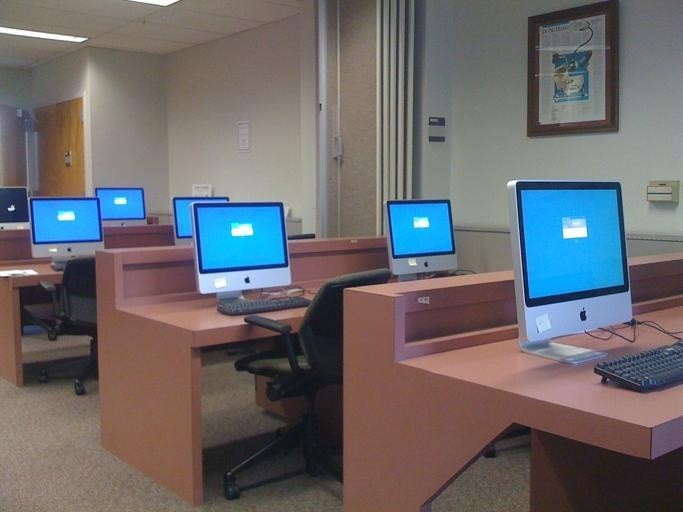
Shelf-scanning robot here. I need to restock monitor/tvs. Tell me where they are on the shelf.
[28,196,105,270]
[190,202,292,302]
[171,197,229,245]
[0,186,29,230]
[95,187,146,226]
[506,178,632,365]
[383,199,458,282]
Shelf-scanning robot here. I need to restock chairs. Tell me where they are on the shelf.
[222,268,392,501]
[21,256,98,395]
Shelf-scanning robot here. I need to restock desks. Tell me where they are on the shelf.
[344,251,683,512]
[0,226,175,387]
[96,236,397,508]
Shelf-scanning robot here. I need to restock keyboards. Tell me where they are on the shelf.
[593,340,683,392]
[217,296,312,315]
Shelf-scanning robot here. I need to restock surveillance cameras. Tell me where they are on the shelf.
[17,108,27,123]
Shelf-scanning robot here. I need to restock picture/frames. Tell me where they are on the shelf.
[527,0,619,138]
